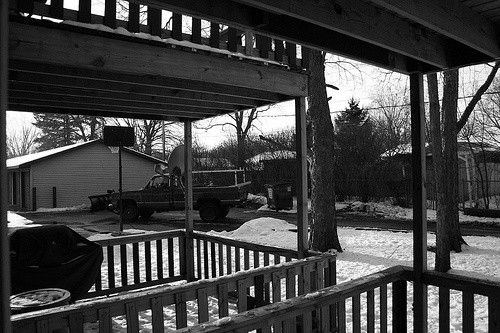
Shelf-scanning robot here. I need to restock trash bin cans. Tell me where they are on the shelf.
[264,183,294,212]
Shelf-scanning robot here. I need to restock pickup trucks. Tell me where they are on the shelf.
[107,167,250,226]
